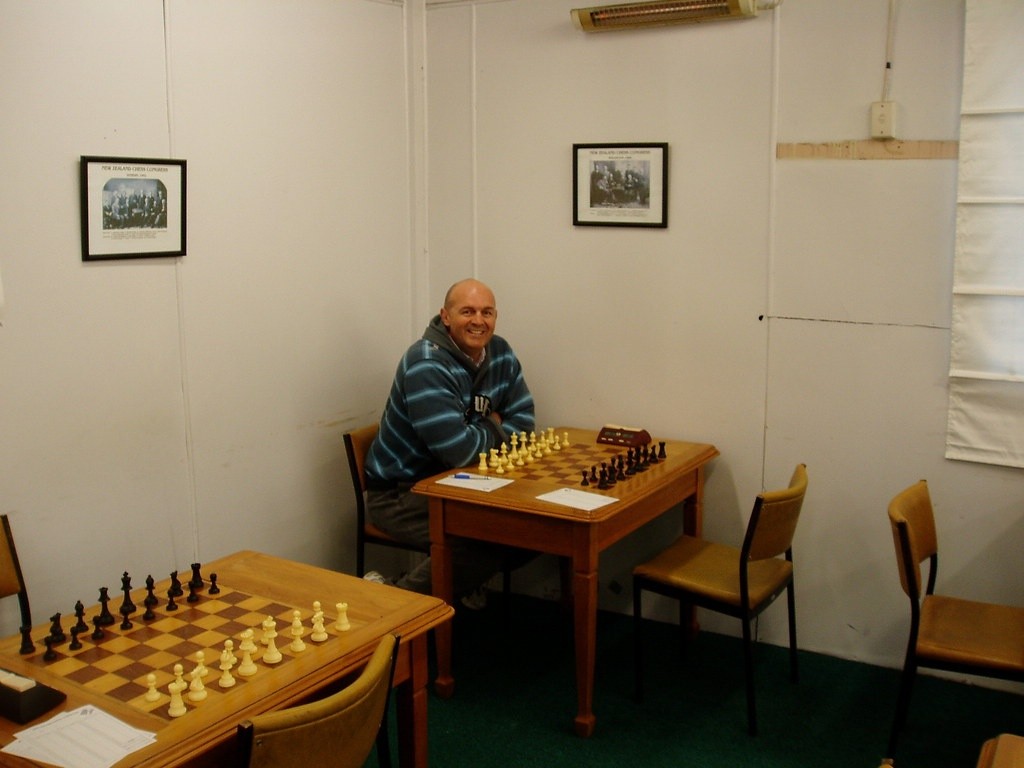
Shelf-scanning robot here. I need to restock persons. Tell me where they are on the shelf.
[591,163,647,205]
[103,189,167,229]
[365,279,535,596]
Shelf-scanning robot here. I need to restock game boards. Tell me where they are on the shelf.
[470,434,675,493]
[13,573,356,723]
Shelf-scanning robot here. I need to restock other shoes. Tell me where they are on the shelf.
[462,575,487,610]
[396,574,431,594]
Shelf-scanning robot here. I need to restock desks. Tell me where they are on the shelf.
[410,427,720,741]
[0,549,455,768]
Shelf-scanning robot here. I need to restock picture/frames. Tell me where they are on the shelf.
[80,155,187,261]
[573,142,668,229]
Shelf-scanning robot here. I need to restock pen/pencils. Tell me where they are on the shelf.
[455,475,491,480]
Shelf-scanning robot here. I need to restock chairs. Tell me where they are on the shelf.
[0,423,512,768]
[630,461,1024,768]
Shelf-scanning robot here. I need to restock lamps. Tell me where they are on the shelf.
[570,0,783,34]
